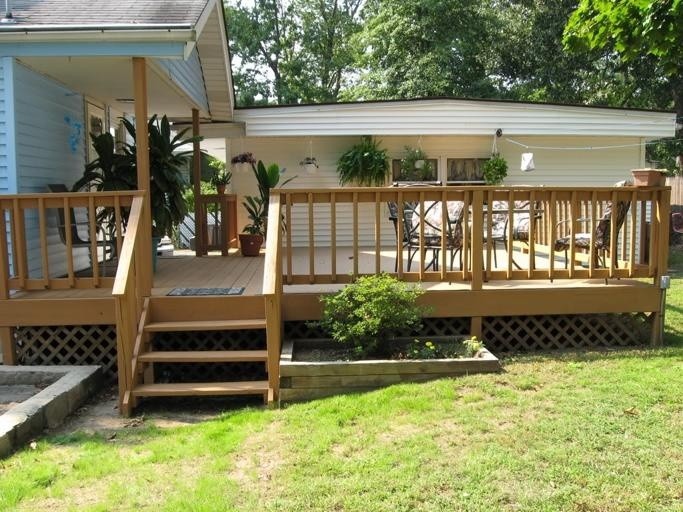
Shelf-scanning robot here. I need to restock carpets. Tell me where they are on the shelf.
[164,287,244,296]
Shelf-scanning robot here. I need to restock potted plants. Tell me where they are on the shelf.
[237,195,268,257]
[278,271,500,403]
[399,143,433,180]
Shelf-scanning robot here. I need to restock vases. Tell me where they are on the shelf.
[631,168,664,187]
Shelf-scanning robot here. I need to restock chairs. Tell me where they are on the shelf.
[387,180,632,284]
[45,182,117,278]
